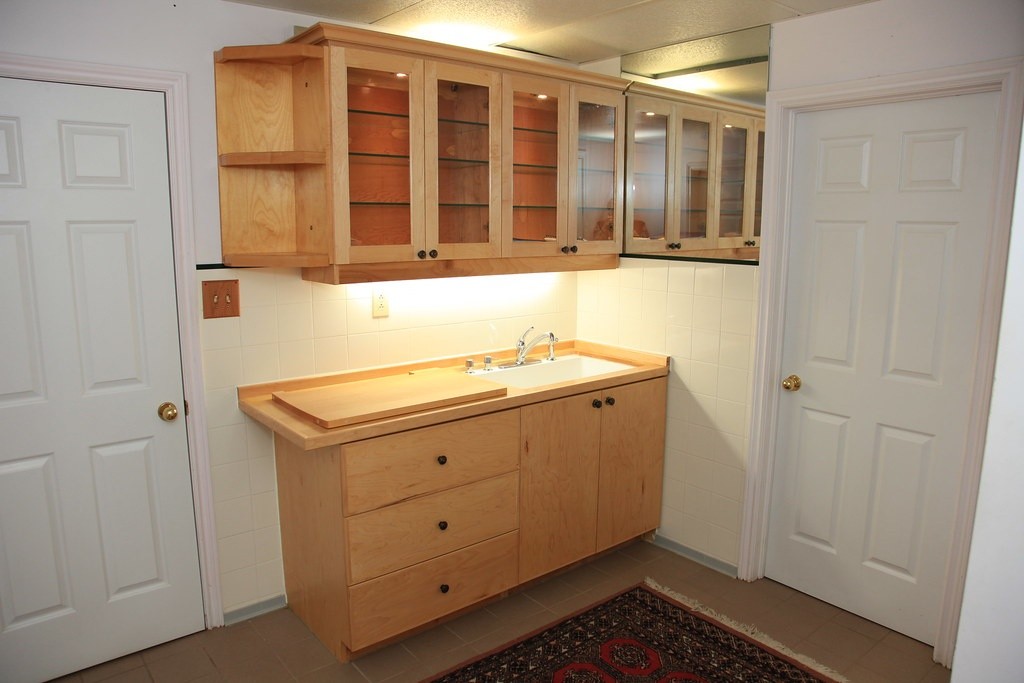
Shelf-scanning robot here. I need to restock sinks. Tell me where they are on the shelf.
[474,354,637,388]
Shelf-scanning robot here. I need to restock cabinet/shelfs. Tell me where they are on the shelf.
[518,376,668,586]
[624,81,718,261]
[213,21,502,285]
[274,406,520,664]
[502,53,635,275]
[713,99,767,259]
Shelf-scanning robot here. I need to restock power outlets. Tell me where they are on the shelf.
[371,293,390,318]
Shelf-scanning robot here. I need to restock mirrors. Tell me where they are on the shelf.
[620,23,772,266]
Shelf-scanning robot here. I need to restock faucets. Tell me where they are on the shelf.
[515,325,555,366]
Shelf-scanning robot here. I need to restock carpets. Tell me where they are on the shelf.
[416,575,852,683]
